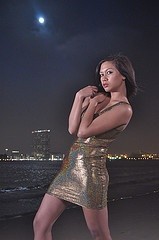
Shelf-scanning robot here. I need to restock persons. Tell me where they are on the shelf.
[32,54,137,236]
